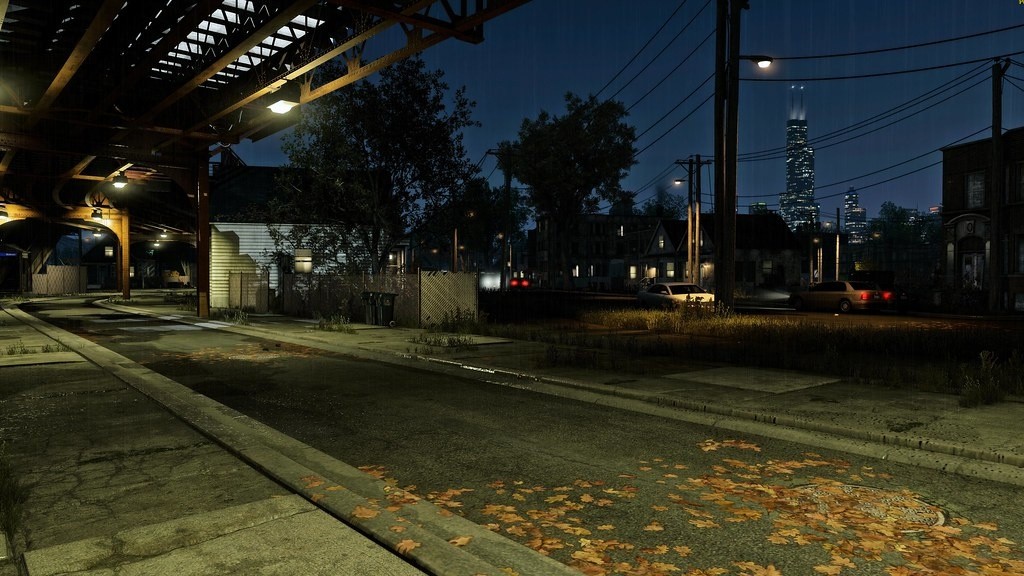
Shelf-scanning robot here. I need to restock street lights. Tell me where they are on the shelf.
[825,221,839,280]
[674,178,692,285]
[814,239,822,282]
[712,52,773,310]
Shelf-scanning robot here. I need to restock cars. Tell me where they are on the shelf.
[788,279,895,315]
[638,284,715,311]
